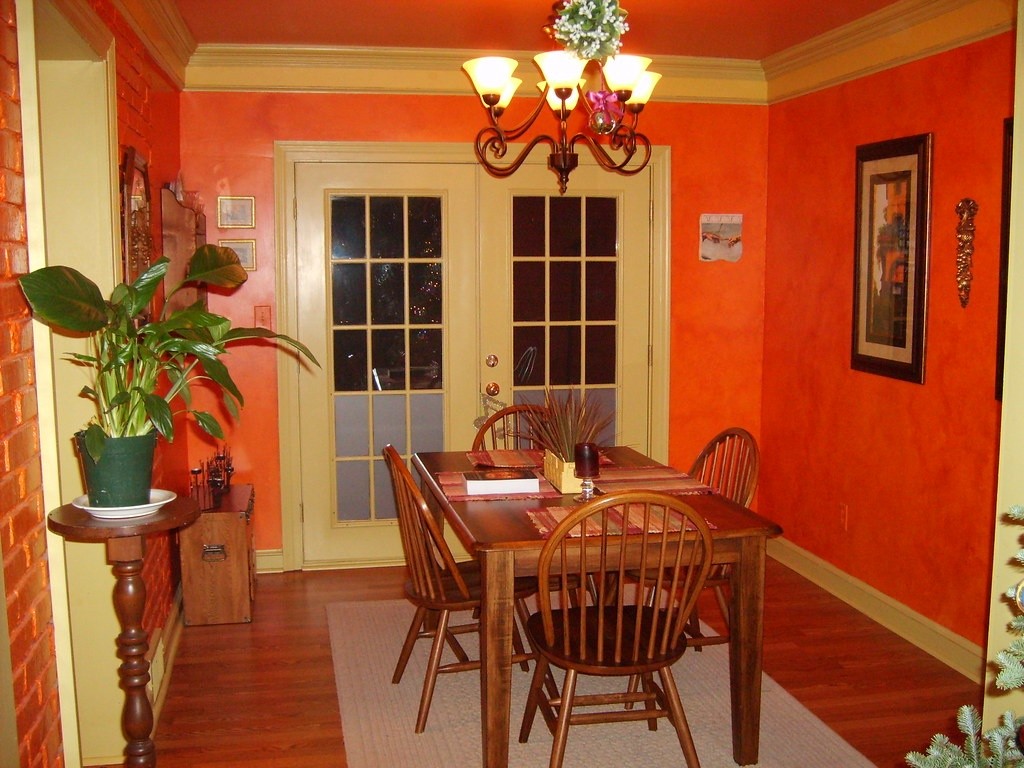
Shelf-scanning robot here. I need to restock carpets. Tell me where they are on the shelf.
[327,581,878,768]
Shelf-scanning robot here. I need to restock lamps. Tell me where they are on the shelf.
[461,25,663,195]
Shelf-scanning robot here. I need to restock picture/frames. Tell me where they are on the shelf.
[219,239,256,271]
[123,148,154,334]
[850,132,933,385]
[218,197,256,228]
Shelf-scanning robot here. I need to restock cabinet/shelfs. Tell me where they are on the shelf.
[178,483,256,628]
[163,171,208,329]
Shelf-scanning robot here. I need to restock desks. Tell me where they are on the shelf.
[409,446,784,768]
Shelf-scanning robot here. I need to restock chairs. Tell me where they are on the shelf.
[473,405,600,609]
[625,426,759,709]
[518,492,714,768]
[384,444,562,734]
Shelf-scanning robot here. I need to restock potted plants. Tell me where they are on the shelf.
[20,243,324,515]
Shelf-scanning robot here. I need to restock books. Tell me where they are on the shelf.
[462,469,541,495]
